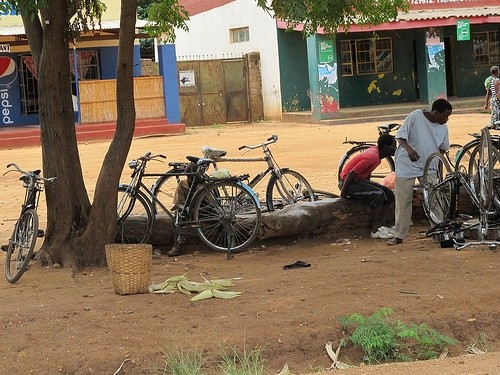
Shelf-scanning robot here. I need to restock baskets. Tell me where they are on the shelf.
[104,243,154,296]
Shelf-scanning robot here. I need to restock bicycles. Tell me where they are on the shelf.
[115,152,262,258]
[1,162,59,283]
[234,188,339,215]
[152,135,316,215]
[422,121,500,251]
[338,123,479,193]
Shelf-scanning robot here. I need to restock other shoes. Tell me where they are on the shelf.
[166,246,184,257]
[370,226,395,239]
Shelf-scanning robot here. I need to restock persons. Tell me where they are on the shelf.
[338,133,397,238]
[484,66,500,130]
[386,98,453,245]
